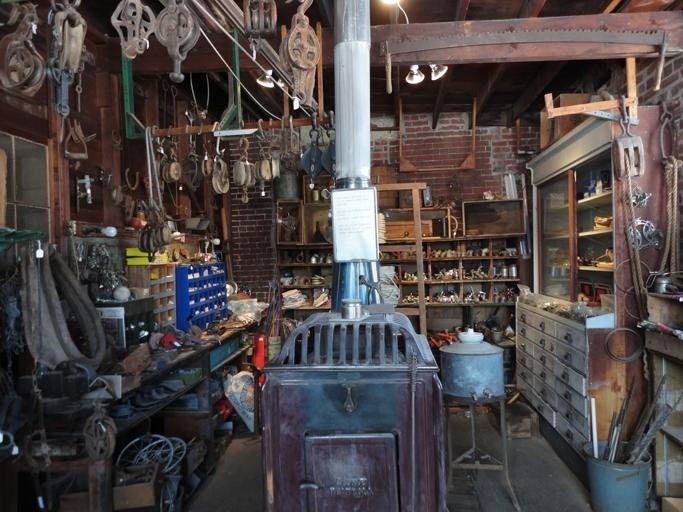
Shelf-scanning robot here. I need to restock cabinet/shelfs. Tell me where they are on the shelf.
[514,104,682,512]
[276,173,525,377]
[1,261,271,511]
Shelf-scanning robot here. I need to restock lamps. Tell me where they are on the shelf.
[405,61,448,85]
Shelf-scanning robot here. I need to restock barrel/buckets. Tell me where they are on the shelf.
[584,440,652,511]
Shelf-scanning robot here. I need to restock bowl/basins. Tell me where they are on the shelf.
[457,331,485,344]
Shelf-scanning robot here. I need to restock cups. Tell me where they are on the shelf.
[489,329,504,343]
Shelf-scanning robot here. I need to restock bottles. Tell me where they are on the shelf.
[500,266,509,279]
[341,298,363,320]
[508,264,518,279]
